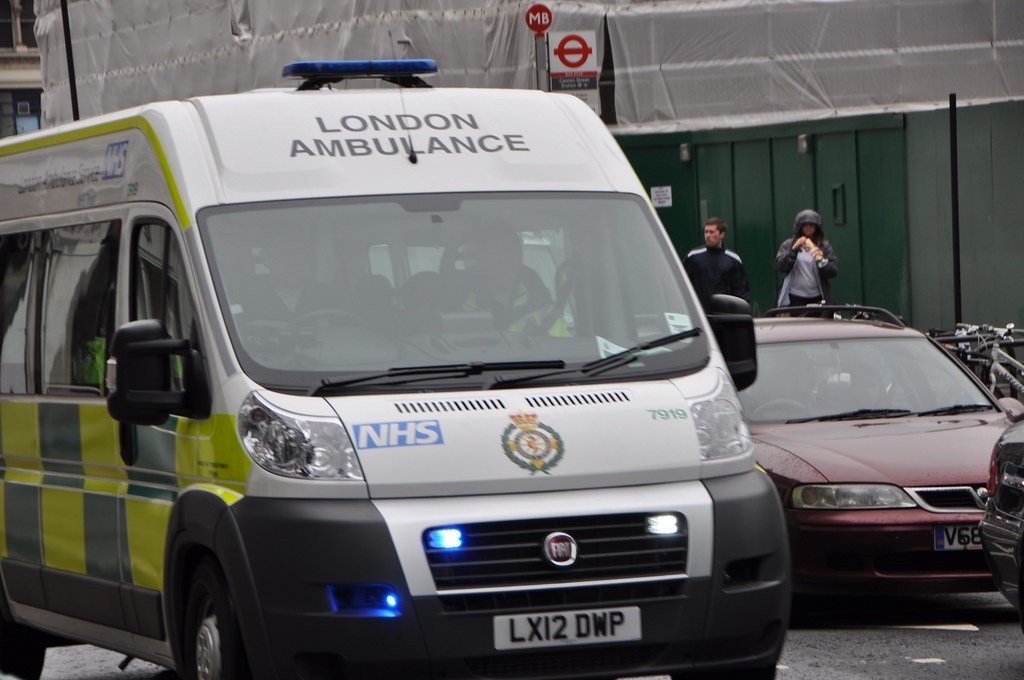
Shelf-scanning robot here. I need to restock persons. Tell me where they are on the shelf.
[464,231,570,338]
[682,217,750,310]
[775,209,839,317]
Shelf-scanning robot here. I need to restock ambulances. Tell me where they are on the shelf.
[3,58,792,680]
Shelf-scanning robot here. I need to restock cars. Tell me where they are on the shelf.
[977,417,1024,639]
[742,306,1024,601]
